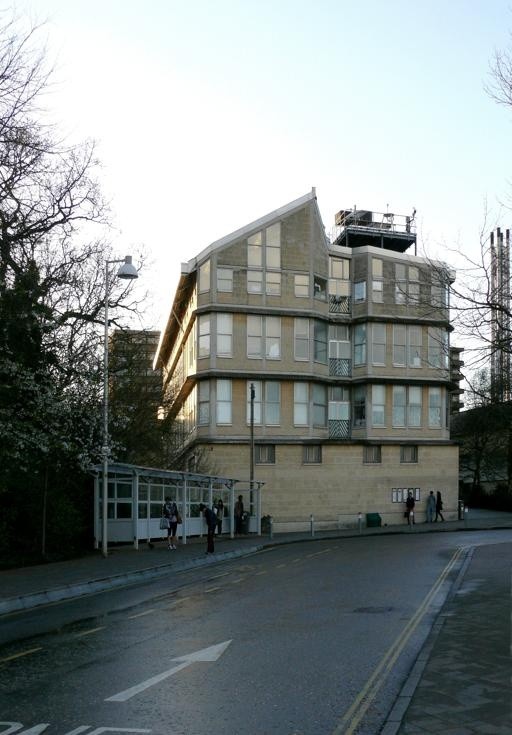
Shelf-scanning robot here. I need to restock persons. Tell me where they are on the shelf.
[424,491,436,523]
[234,494,243,536]
[163,496,178,551]
[216,499,224,537]
[432,491,445,523]
[405,492,416,525]
[199,503,219,555]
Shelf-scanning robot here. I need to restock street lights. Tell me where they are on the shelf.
[101,255,138,554]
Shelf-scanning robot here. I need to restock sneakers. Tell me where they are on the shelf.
[168,544,176,550]
[434,519,445,522]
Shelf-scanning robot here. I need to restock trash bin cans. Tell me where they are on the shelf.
[261,515,272,536]
[240,511,250,534]
[458,500,464,519]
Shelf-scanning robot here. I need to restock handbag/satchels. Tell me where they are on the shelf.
[404,512,408,518]
[159,518,170,529]
